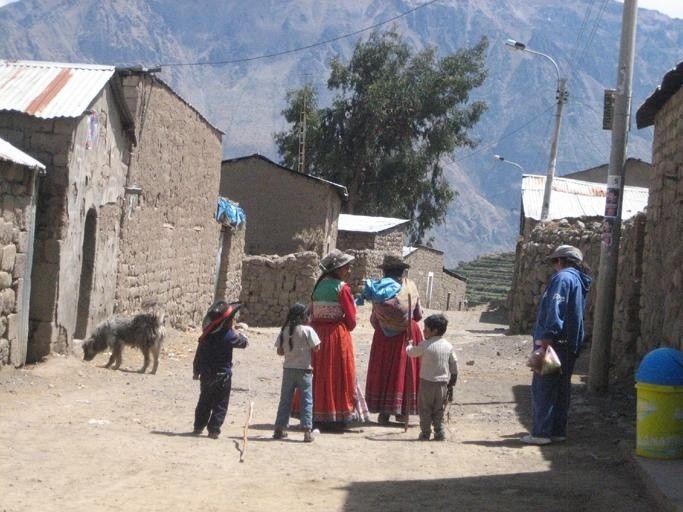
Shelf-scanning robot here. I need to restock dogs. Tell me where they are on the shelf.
[81,309,167,375]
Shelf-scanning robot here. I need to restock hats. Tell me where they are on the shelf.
[376,255,410,269]
[546,245,583,262]
[319,249,355,273]
[202,301,243,335]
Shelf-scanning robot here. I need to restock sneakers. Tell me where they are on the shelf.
[435,431,444,441]
[552,437,567,441]
[208,430,219,438]
[378,414,390,424]
[520,435,551,444]
[274,430,287,438]
[419,432,429,441]
[193,426,203,433]
[304,428,320,442]
[396,414,408,422]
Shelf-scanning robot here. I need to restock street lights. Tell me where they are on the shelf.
[504,36,566,222]
[492,152,526,175]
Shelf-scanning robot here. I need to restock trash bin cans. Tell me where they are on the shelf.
[633,347,683,460]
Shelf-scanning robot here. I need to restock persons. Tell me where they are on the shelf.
[289,248,358,427]
[354,253,424,426]
[190,300,250,439]
[519,244,592,446]
[271,303,322,442]
[405,311,459,441]
